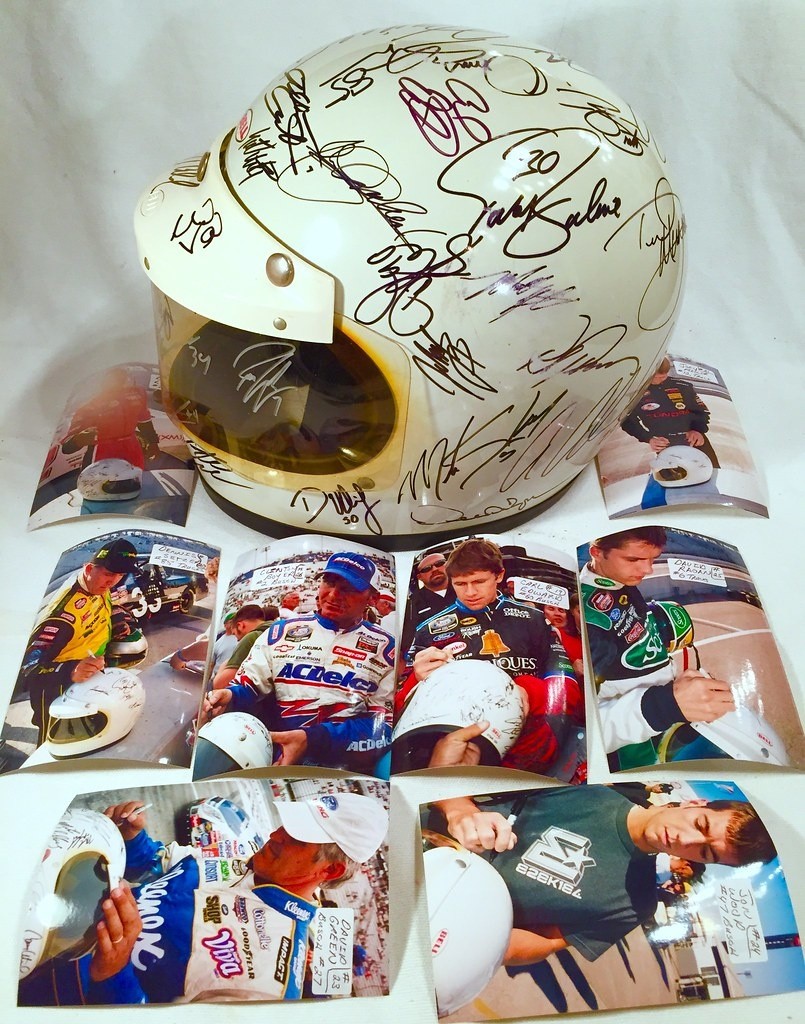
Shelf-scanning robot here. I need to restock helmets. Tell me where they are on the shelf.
[390,659,524,776]
[19,809,126,979]
[76,458,142,501]
[651,445,712,487]
[135,23,687,551]
[192,712,273,783]
[690,704,790,766]
[46,667,145,760]
[423,846,513,1020]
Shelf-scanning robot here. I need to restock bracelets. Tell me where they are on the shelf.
[178,649,187,662]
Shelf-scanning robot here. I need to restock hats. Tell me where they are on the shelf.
[223,612,238,625]
[317,552,379,591]
[93,538,140,573]
[273,792,389,864]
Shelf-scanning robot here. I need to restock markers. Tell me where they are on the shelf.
[206,679,214,723]
[487,792,529,866]
[698,667,717,691]
[652,435,670,446]
[115,802,155,828]
[412,644,455,663]
[86,648,106,675]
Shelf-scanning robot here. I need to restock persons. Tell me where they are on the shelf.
[429,720,490,769]
[311,778,389,996]
[170,557,220,670]
[620,357,723,468]
[520,593,584,677]
[420,781,776,966]
[200,552,396,766]
[396,540,581,772]
[17,792,388,1006]
[20,538,138,749]
[398,553,458,675]
[209,550,395,693]
[608,781,706,944]
[62,366,160,468]
[580,526,736,773]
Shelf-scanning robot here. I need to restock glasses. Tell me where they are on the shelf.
[418,559,446,574]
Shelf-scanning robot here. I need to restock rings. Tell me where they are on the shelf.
[113,936,123,944]
